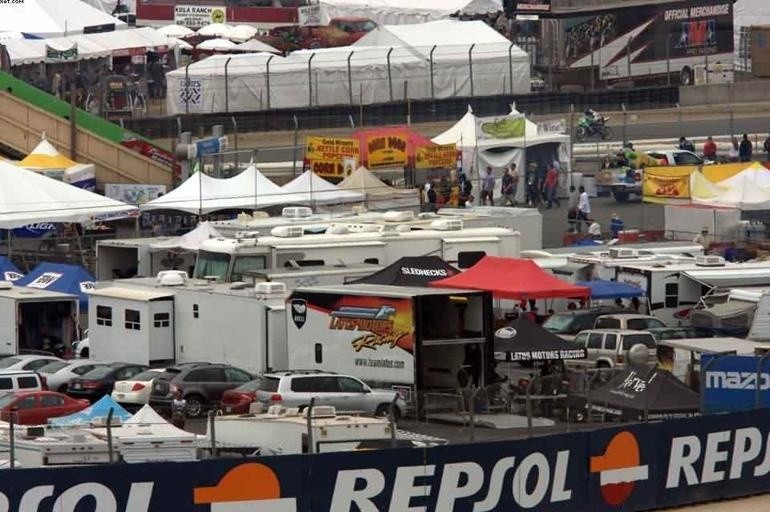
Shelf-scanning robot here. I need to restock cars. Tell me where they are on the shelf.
[112,13,137,30]
[529,74,546,92]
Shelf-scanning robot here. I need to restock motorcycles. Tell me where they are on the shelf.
[575,116,614,141]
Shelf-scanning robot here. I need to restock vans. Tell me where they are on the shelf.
[543,295,755,383]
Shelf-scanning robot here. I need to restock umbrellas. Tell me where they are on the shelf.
[156,22,284,63]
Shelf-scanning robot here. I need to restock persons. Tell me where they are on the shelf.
[418,162,562,210]
[610,213,624,239]
[574,186,591,229]
[566,186,578,231]
[600,140,641,174]
[518,296,641,321]
[170,388,187,430]
[678,136,696,153]
[587,217,602,238]
[151,57,164,99]
[703,136,716,157]
[739,133,753,162]
[585,107,604,132]
[692,226,709,252]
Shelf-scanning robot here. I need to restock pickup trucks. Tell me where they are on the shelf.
[596,150,720,202]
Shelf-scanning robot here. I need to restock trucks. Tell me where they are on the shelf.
[38,221,116,253]
[270,15,379,47]
[194,225,522,283]
